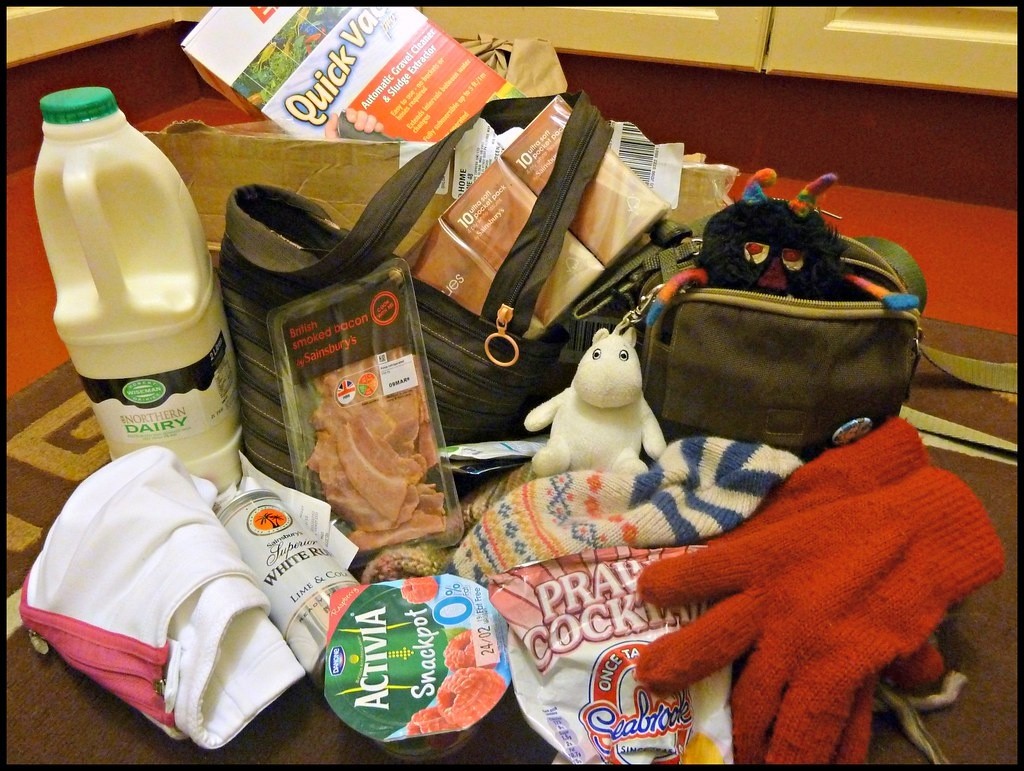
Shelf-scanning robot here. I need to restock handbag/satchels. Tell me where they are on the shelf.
[218,87,613,500]
[574,214,1017,457]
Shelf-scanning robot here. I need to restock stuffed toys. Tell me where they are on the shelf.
[523,319,667,476]
[647,167,920,328]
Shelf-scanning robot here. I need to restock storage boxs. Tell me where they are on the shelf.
[181,7,504,143]
[142,118,455,298]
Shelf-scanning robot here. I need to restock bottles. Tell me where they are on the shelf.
[34,87,240,496]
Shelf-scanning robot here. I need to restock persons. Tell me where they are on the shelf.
[324,109,384,140]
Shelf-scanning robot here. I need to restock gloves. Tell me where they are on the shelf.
[636,416,1004,763]
